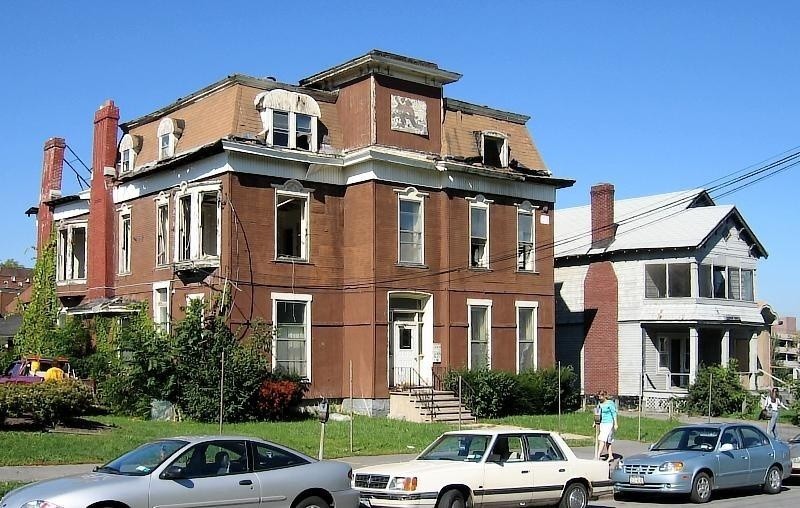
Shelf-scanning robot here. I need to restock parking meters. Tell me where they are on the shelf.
[594,406,602,458]
[317,400,329,459]
[765,402,773,435]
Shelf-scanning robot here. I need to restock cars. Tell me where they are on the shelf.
[347,425,612,508]
[607,419,792,505]
[0,432,362,507]
[786,432,800,476]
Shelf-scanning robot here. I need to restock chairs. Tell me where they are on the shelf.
[184,448,208,478]
[213,451,233,473]
[724,432,737,449]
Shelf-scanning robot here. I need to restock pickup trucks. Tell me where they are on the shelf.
[1,356,96,389]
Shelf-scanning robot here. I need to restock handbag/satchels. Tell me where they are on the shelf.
[594,408,602,423]
[758,405,773,420]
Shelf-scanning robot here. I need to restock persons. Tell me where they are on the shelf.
[593,390,619,463]
[43,358,64,380]
[764,386,790,442]
